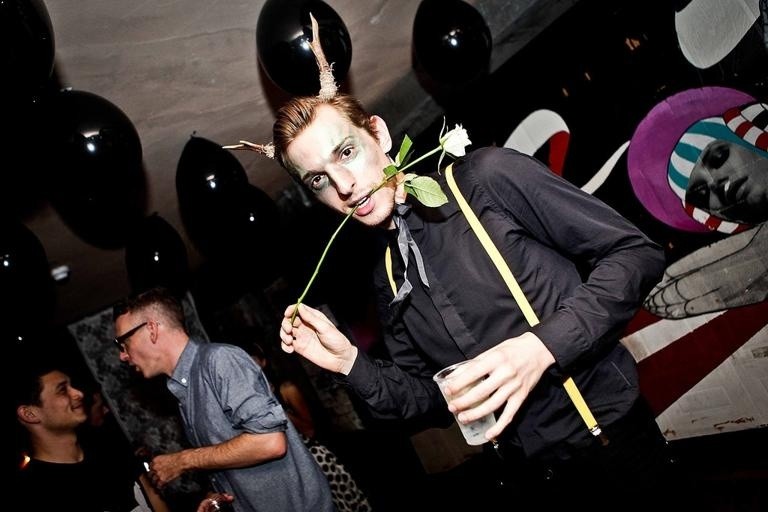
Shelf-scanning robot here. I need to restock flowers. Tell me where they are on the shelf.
[289,117,472,327]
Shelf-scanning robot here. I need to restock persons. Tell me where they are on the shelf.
[246,351,375,512]
[683,141,766,218]
[271,89,670,511]
[0,367,240,512]
[79,387,112,431]
[114,286,336,512]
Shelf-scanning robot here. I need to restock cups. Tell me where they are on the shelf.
[432,356,505,447]
[206,497,234,512]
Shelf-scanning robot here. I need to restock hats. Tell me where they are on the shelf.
[627,86,757,234]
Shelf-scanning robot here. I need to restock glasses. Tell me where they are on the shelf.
[113,322,147,352]
